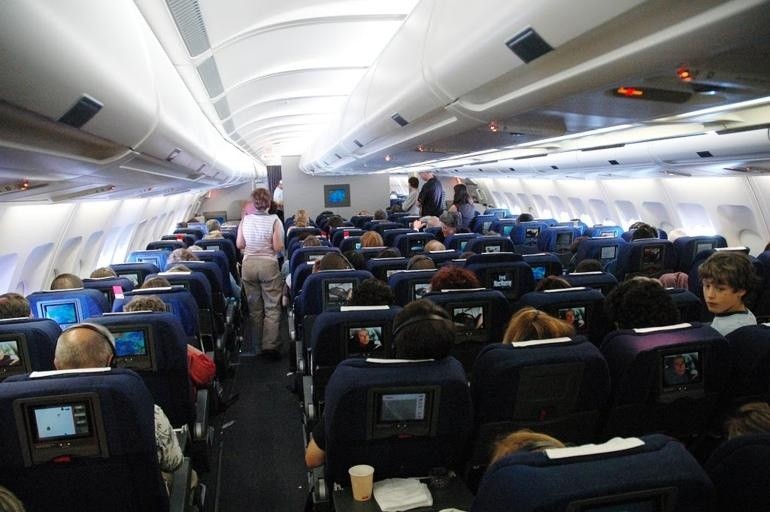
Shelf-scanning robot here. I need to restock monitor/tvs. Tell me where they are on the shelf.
[0,213,226,469]
[324,184,350,208]
[299,212,715,443]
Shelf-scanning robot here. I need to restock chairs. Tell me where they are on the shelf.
[0,203,235,512]
[288,205,767,510]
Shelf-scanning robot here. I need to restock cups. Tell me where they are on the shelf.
[348,464,375,502]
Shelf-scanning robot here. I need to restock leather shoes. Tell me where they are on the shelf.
[215,390,239,417]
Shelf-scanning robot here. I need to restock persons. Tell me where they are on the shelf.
[286,210,690,305]
[725,401,770,443]
[234,189,285,361]
[603,280,681,333]
[1,486,25,511]
[272,178,284,211]
[449,176,476,230]
[488,429,565,465]
[401,176,420,213]
[268,200,283,226]
[54,323,183,499]
[0,219,241,404]
[503,306,576,346]
[240,192,261,218]
[415,169,446,216]
[304,299,456,469]
[697,250,757,337]
